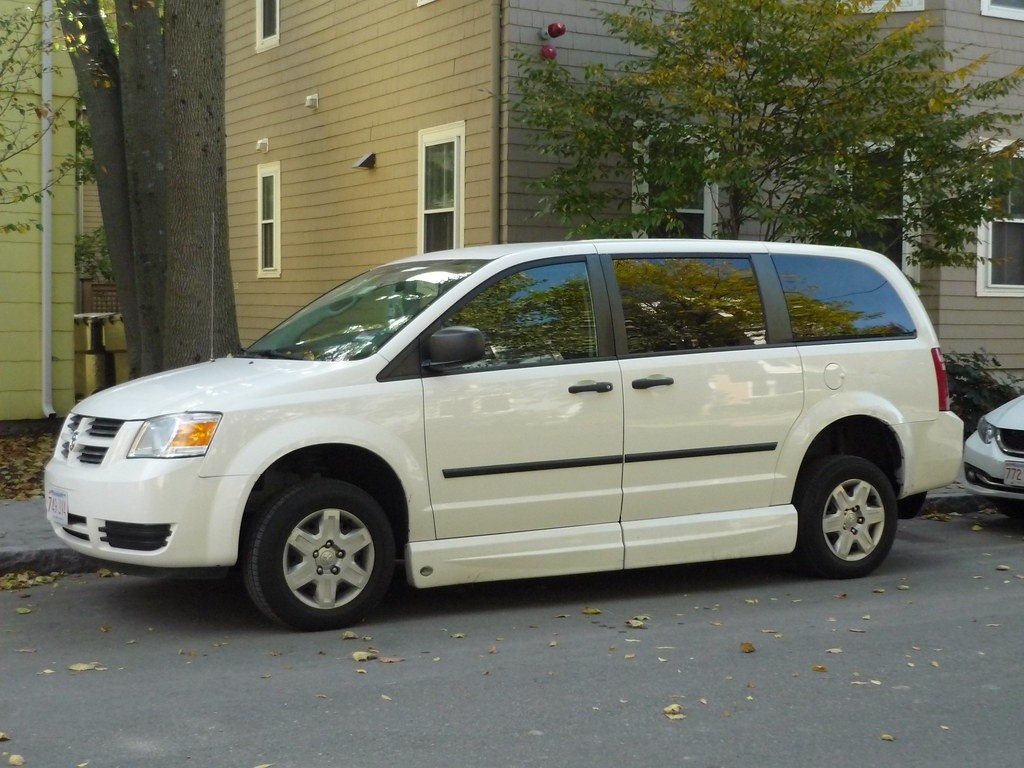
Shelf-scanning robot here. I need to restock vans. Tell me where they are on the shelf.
[44,238,965,631]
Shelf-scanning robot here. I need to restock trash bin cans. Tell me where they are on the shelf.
[73,311,131,402]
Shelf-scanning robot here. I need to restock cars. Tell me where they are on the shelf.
[964,392,1024,519]
